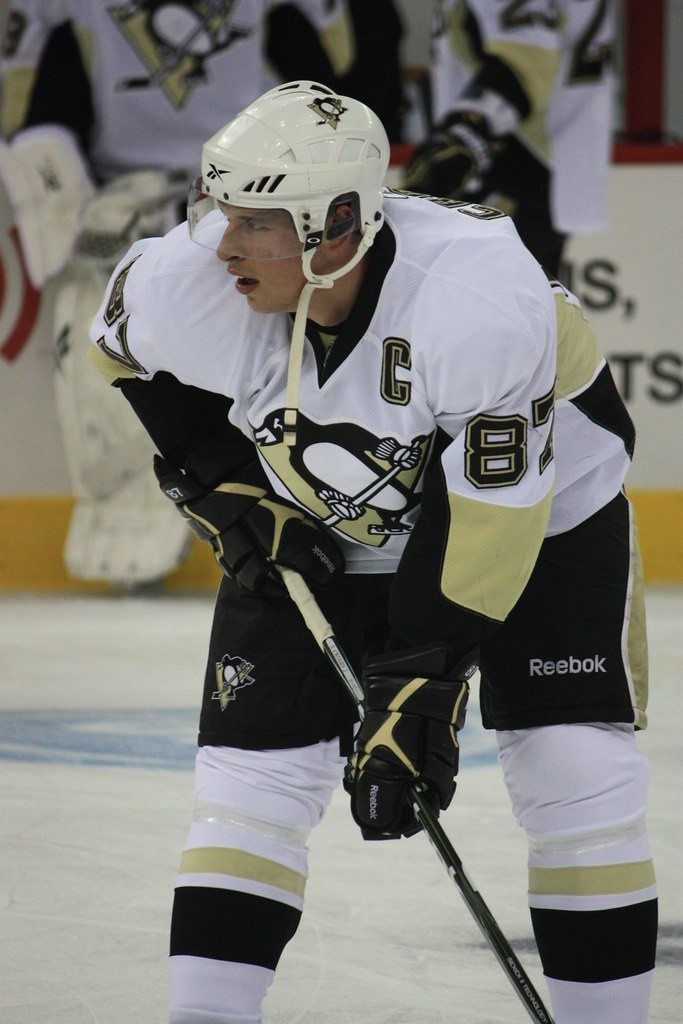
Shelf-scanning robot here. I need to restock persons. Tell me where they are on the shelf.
[88,79,659,1024]
[403,3,624,289]
[4,1,410,589]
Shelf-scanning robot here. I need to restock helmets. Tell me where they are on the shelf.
[201,81,390,243]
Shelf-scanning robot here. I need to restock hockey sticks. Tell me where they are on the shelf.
[259,550,573,1024]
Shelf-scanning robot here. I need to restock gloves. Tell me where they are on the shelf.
[176,460,347,598]
[343,640,470,840]
[400,110,501,202]
